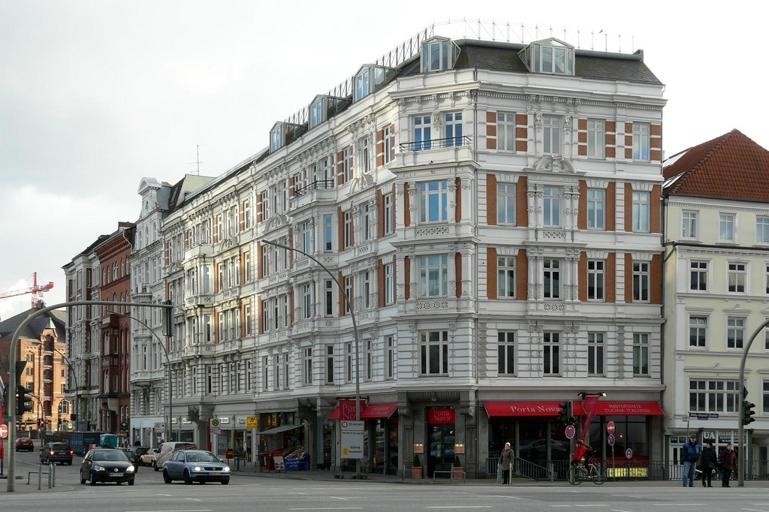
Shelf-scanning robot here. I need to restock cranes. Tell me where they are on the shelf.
[0,270,55,309]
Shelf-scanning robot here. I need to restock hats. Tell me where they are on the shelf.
[689,434,697,438]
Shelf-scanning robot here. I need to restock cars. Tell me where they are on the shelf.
[510,437,569,460]
[78,447,136,486]
[15,437,35,453]
[38,441,74,466]
[161,448,232,485]
[138,447,161,468]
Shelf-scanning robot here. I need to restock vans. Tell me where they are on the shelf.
[151,441,197,472]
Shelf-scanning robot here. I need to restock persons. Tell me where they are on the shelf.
[497,442,514,484]
[681,433,736,487]
[571,441,588,466]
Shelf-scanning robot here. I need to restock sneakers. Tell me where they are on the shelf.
[586,473,591,476]
[683,484,694,487]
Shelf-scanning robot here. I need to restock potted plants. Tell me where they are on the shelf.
[451,456,465,479]
[408,455,422,479]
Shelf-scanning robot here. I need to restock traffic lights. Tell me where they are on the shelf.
[741,400,757,426]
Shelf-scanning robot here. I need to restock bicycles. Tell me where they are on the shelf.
[565,456,608,485]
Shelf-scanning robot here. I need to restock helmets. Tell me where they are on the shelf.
[576,440,583,444]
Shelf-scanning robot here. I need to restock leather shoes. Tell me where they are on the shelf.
[502,482,512,484]
[703,485,712,487]
[722,484,731,487]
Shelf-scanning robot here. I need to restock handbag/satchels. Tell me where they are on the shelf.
[708,463,715,468]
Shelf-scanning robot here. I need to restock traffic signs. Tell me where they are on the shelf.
[606,420,616,435]
